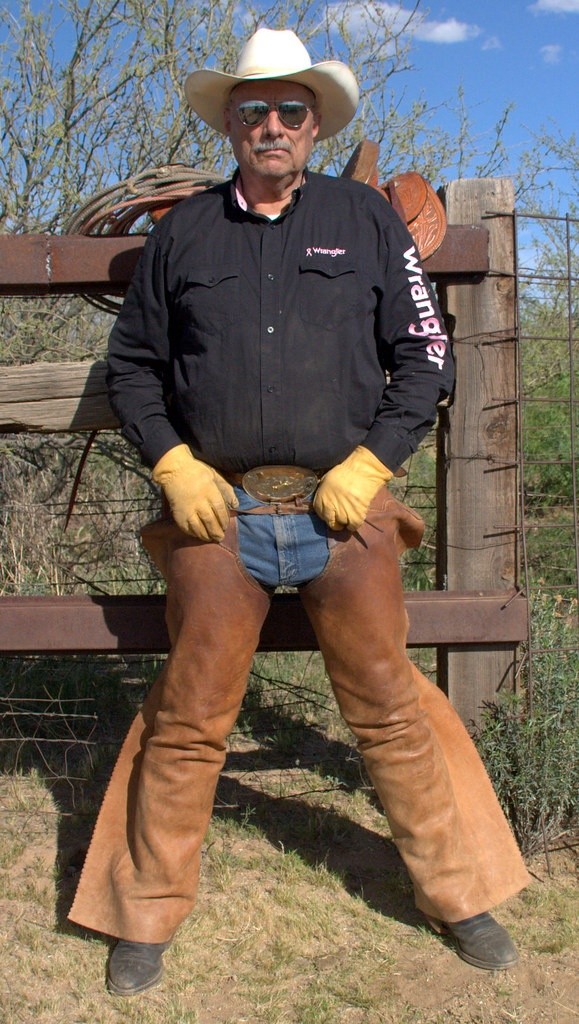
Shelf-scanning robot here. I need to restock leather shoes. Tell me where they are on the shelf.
[106,934,174,996]
[426,912,518,970]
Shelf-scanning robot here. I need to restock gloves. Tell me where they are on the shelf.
[312,444,394,532]
[151,443,239,542]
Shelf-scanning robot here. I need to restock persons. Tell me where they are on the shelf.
[67,26,531,996]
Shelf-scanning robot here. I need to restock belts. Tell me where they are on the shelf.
[223,465,318,515]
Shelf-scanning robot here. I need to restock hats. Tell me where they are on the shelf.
[184,28,359,141]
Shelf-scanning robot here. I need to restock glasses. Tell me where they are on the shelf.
[226,98,315,130]
